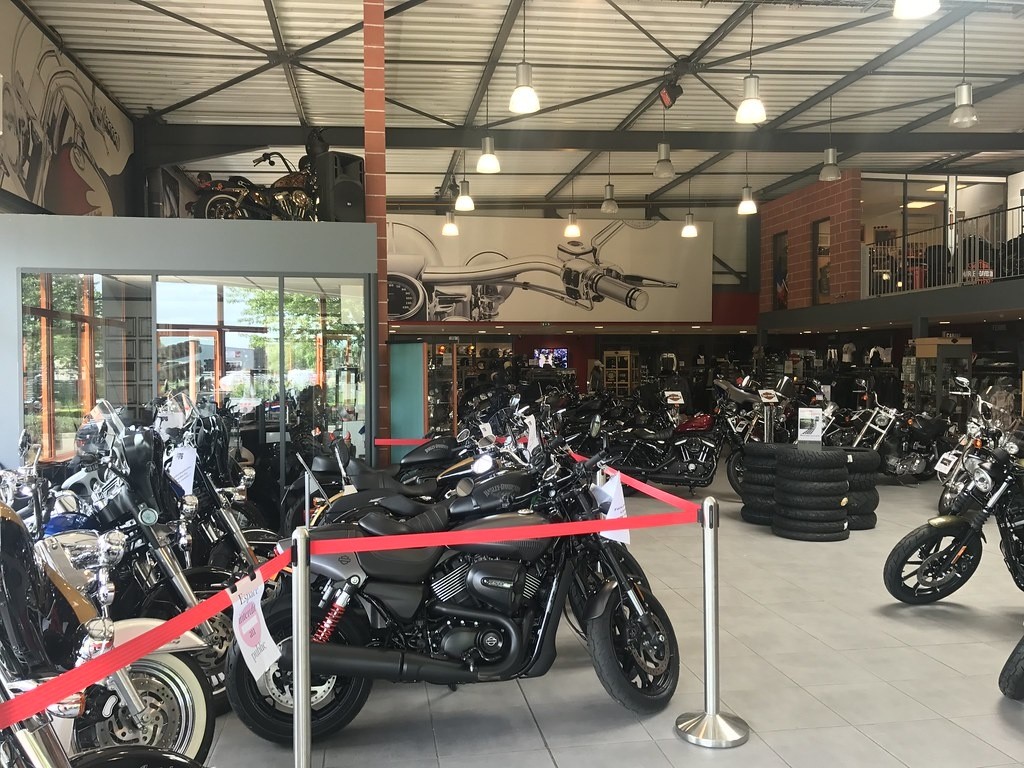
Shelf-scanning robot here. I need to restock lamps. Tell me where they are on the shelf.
[564,177,581,237]
[681,178,698,237]
[659,84,684,109]
[442,175,460,237]
[476,85,501,173]
[948,16,980,129]
[734,7,766,124]
[818,94,842,182]
[653,106,675,179]
[454,149,475,211]
[737,151,758,215]
[509,0,541,113]
[600,151,618,214]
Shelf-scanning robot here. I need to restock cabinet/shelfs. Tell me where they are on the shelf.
[603,350,633,397]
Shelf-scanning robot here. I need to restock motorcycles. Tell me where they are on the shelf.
[193,124,330,221]
[0,352,1024,767]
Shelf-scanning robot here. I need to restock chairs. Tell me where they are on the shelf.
[924,244,954,285]
